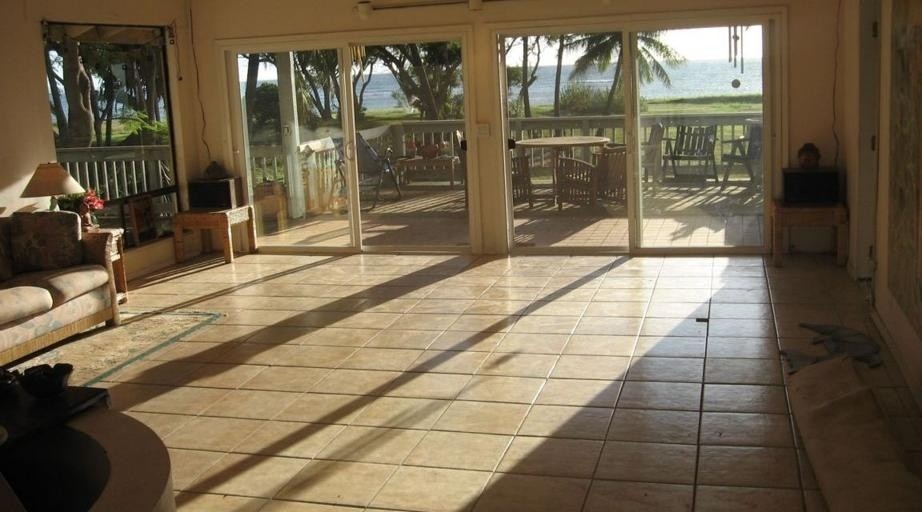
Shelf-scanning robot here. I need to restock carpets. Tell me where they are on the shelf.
[3,309,229,389]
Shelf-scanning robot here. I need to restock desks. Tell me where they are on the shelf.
[0,405,181,512]
[80,225,130,304]
[396,156,463,191]
[166,203,261,265]
[769,196,850,271]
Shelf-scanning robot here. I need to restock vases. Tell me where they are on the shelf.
[76,210,93,227]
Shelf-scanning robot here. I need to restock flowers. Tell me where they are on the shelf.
[55,184,108,215]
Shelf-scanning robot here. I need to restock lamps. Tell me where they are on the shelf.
[18,159,86,212]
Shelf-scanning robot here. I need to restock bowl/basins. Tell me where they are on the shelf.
[18,362,73,401]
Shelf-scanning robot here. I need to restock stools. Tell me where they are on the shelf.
[251,181,289,234]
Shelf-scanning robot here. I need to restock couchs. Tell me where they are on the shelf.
[1,213,122,373]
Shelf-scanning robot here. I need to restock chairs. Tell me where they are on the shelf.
[639,118,769,197]
[330,133,402,213]
[509,136,629,215]
